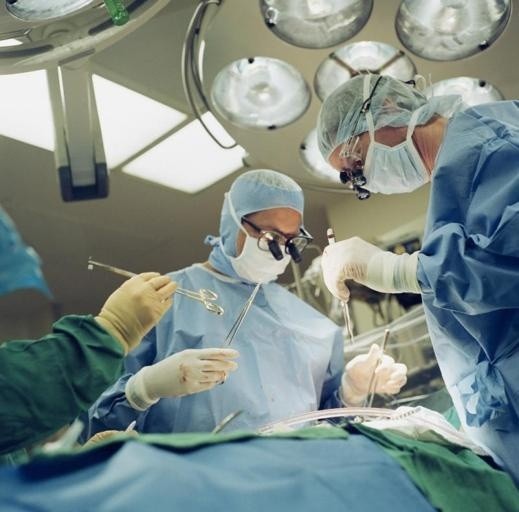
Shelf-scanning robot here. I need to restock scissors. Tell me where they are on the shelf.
[87,260,225,316]
[214,283,261,385]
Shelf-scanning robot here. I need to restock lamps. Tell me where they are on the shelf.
[179,1,518,202]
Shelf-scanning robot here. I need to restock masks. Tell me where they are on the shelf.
[230,235,292,287]
[353,139,431,196]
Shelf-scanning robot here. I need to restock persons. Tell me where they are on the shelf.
[69,168,407,445]
[0,271,178,466]
[315,75,519,488]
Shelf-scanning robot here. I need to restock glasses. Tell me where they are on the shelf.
[242,216,313,257]
